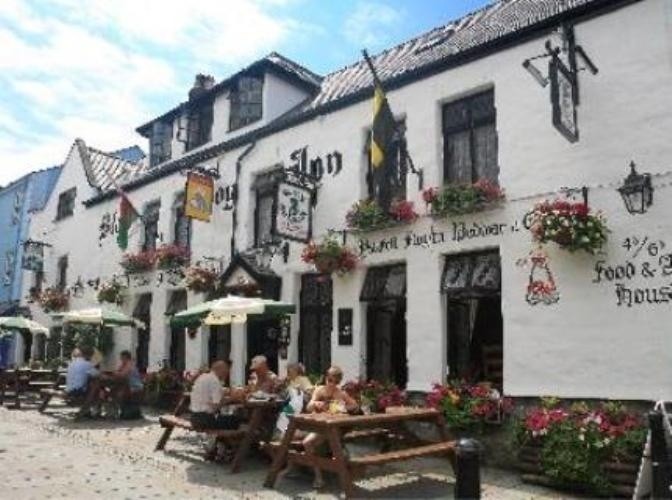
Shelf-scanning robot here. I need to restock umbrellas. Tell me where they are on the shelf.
[170,291,297,386]
[0,316,49,364]
[62,306,146,349]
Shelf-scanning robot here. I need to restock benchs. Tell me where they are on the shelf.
[259,435,465,500]
[156,412,251,473]
[1,367,125,422]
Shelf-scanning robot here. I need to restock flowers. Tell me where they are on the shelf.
[424,378,646,495]
[340,173,507,227]
[98,243,218,304]
[521,197,611,307]
[301,240,358,279]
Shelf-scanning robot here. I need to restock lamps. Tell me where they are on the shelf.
[616,159,653,215]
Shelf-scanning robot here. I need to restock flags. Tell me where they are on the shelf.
[117,197,138,250]
[372,82,399,187]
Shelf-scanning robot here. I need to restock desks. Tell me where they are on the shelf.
[262,406,443,500]
[155,390,287,473]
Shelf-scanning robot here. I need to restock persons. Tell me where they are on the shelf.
[71,346,103,368]
[257,361,314,464]
[247,354,278,393]
[299,366,358,492]
[188,359,236,464]
[64,344,104,420]
[105,350,145,420]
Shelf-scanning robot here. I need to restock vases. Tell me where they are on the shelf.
[313,254,339,275]
